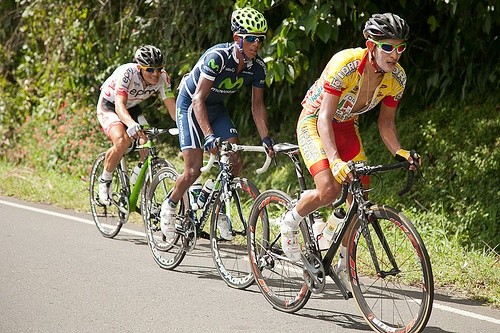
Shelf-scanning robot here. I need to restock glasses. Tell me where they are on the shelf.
[366,38,409,55]
[234,33,267,43]
[138,64,163,72]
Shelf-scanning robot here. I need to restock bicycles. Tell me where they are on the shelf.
[145,138,271,290]
[88,125,185,251]
[247,149,434,333]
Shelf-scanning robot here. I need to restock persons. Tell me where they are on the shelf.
[281,13,422,293]
[161,7,278,241]
[97,45,176,206]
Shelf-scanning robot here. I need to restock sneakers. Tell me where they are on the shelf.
[160,198,177,239]
[215,211,236,241]
[280,210,300,261]
[333,263,366,294]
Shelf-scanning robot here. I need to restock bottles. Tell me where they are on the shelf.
[322,207,346,242]
[197,178,215,208]
[129,163,143,187]
[189,174,202,211]
[312,211,330,251]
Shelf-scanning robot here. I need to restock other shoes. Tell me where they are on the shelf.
[98,175,113,206]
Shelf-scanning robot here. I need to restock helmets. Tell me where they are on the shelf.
[230,7,268,35]
[362,12,410,42]
[135,45,164,67]
